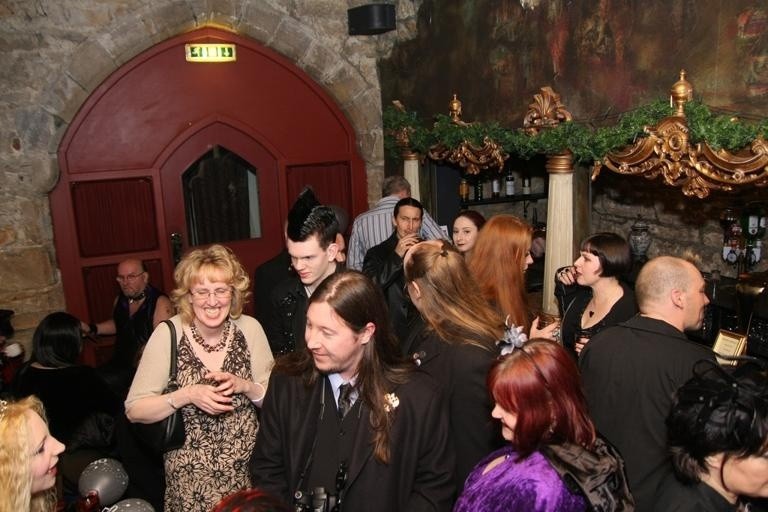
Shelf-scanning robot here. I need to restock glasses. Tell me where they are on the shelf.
[403,280,410,300]
[116,272,144,282]
[188,288,232,299]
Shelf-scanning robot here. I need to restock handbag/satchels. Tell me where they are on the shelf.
[127,383,186,459]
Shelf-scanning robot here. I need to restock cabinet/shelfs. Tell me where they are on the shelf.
[429,155,549,294]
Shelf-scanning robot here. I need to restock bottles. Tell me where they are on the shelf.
[505,166,515,196]
[492,179,500,197]
[458,178,470,202]
[473,183,483,201]
[523,175,531,194]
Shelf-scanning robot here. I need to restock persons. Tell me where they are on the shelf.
[2,174,768,512]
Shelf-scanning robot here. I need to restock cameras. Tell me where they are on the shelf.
[294,486,341,512]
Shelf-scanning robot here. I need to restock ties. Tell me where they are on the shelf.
[338,384,353,421]
